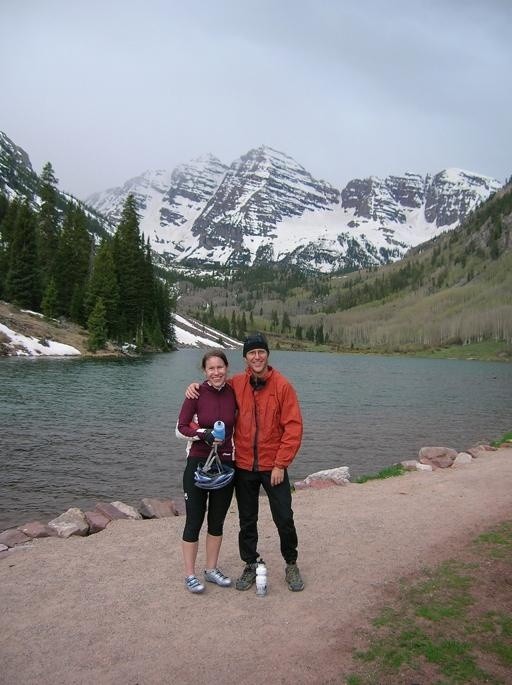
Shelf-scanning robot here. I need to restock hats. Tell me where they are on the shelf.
[243,335,270,358]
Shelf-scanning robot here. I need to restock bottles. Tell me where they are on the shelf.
[214,421,226,441]
[256,558,267,597]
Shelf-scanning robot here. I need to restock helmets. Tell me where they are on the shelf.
[194,462,235,490]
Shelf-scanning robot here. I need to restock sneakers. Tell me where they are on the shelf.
[286,564,304,591]
[185,575,205,594]
[204,568,232,587]
[236,563,258,590]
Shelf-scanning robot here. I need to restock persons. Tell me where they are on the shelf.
[185,334,306,592]
[173,349,239,594]
